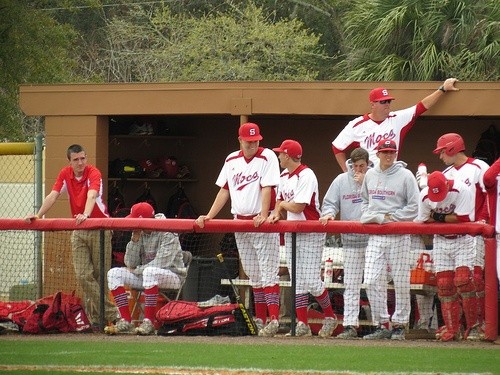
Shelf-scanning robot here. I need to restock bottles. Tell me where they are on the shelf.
[416,260,424,269]
[417,163,427,189]
[425,260,432,272]
[324,258,333,283]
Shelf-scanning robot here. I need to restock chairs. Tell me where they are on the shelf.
[131,251,192,336]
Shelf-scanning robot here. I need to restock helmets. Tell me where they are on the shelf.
[433,133,466,157]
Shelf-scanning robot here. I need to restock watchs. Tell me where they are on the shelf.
[439,85,447,93]
[83,213,90,218]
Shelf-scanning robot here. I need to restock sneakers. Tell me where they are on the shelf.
[285,321,312,337]
[388,325,406,341]
[318,313,338,338]
[134,318,156,335]
[103,318,135,334]
[362,324,392,340]
[336,325,359,339]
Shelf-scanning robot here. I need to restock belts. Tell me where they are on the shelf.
[237,213,257,220]
[441,234,463,240]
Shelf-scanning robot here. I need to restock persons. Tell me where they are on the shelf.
[319,148,375,342]
[418,170,481,340]
[359,139,419,340]
[195,122,281,337]
[23,144,121,329]
[416,133,491,341]
[103,203,188,335]
[330,78,460,174]
[267,140,340,338]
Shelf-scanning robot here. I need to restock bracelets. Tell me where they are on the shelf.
[132,238,139,242]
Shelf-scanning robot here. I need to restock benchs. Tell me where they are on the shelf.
[221,245,440,340]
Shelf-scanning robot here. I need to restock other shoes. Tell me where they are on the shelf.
[255,318,263,331]
[465,326,481,340]
[264,319,280,335]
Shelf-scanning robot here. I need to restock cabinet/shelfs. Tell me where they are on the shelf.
[108,134,197,189]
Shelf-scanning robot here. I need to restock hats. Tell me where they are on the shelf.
[427,170,449,202]
[272,139,303,159]
[368,88,396,102]
[378,139,398,152]
[124,202,155,218]
[238,123,263,141]
